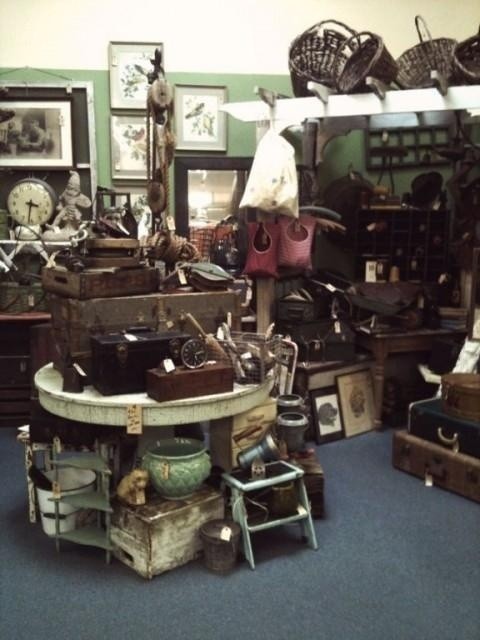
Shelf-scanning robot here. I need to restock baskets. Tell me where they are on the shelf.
[214,330,282,385]
[288,16,480,98]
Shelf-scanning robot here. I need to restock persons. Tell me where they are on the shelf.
[17,112,47,154]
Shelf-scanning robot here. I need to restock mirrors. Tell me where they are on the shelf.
[173,155,256,263]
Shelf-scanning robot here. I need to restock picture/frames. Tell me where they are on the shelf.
[110,111,157,180]
[309,383,345,446]
[0,96,76,171]
[108,40,164,114]
[172,84,228,153]
[334,366,383,441]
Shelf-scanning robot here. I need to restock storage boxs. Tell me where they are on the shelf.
[45,290,241,380]
[41,262,157,298]
[100,482,226,579]
[89,329,195,397]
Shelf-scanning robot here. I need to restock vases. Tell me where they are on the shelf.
[142,436,213,501]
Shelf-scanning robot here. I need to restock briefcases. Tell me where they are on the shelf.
[407,397,480,458]
[392,431,480,504]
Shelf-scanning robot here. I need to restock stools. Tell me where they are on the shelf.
[221,460,318,569]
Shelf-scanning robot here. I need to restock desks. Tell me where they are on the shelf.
[353,320,469,431]
[34,357,276,493]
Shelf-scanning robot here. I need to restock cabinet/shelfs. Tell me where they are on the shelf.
[354,204,450,285]
[207,397,279,472]
[47,443,117,565]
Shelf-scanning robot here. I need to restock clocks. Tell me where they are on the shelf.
[181,340,209,370]
[7,170,57,228]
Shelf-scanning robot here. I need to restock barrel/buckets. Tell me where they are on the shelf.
[30,467,97,538]
